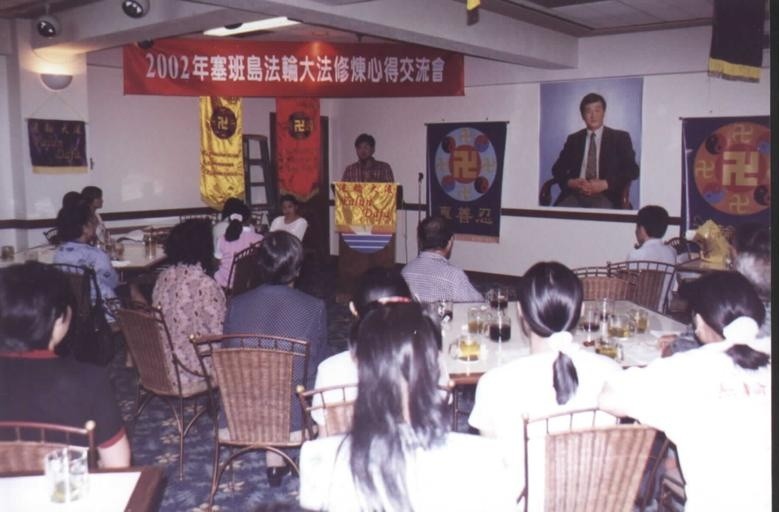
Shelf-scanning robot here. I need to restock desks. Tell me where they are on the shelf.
[2,465,166,509]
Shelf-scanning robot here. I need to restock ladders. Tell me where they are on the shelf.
[244,134,273,208]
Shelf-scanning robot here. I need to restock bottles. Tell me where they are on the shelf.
[92,227,126,260]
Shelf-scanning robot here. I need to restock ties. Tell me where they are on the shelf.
[585,131,598,182]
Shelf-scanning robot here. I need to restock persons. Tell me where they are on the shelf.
[552,92,639,209]
[50,186,309,369]
[220,231,331,485]
[342,134,394,182]
[2,260,132,474]
[400,215,483,304]
[301,263,771,512]
[625,204,678,314]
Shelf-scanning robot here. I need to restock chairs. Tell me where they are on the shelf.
[293,383,458,442]
[539,173,632,208]
[190,331,313,512]
[105,294,225,480]
[521,406,673,510]
[3,416,97,476]
[1,208,695,383]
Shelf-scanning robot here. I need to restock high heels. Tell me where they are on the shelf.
[265,462,292,489]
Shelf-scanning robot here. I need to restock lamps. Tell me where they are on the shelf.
[40,74,74,90]
[121,1,150,20]
[35,4,62,38]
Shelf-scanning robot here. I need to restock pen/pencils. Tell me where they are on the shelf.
[680,332,696,336]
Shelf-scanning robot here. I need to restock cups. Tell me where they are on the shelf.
[142,225,157,257]
[577,297,649,359]
[1,246,15,262]
[22,250,38,264]
[433,284,513,364]
[44,443,92,503]
